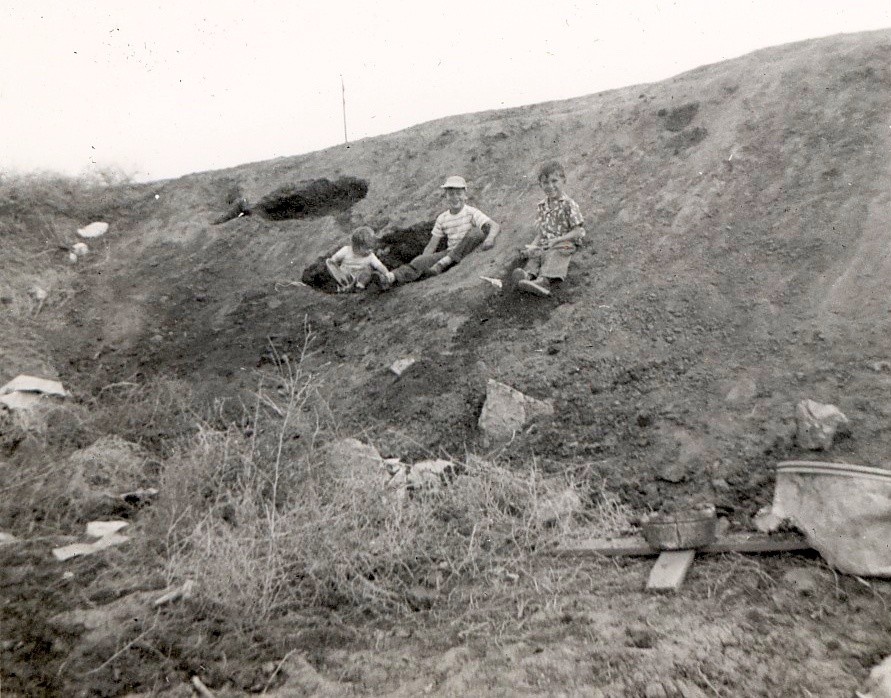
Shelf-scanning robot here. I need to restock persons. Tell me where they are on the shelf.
[512,159,586,298]
[325,226,389,293]
[371,176,500,290]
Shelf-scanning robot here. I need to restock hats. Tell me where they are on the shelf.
[441,176,467,191]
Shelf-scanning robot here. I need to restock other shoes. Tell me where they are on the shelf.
[372,271,389,291]
[339,274,355,293]
[512,267,530,294]
[355,283,364,293]
[430,262,444,276]
[519,277,550,297]
